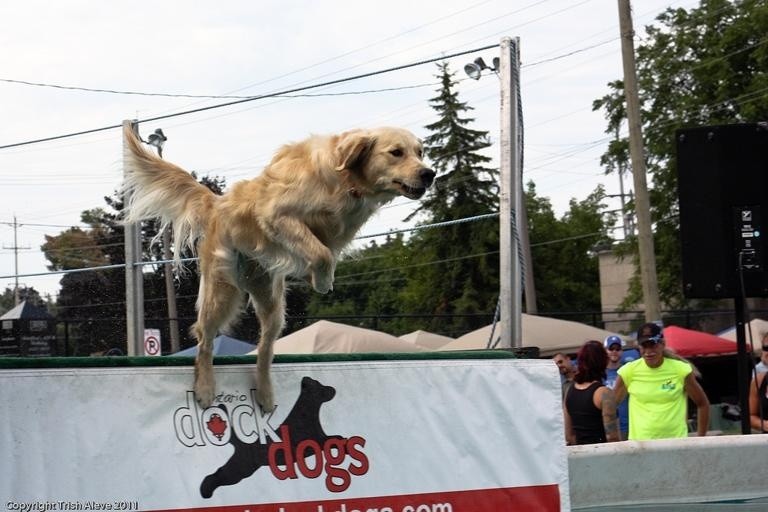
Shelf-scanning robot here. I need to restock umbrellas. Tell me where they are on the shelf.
[663,324,754,359]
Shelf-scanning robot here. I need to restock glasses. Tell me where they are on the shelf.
[608,346,622,351]
[762,345,767,352]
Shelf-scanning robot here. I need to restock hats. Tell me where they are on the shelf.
[636,323,664,345]
[607,336,621,346]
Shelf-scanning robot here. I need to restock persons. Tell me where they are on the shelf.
[599,335,628,440]
[752,331,768,377]
[552,352,579,384]
[749,371,768,433]
[612,321,710,440]
[564,339,619,445]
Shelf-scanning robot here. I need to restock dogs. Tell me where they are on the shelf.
[109,120,437,415]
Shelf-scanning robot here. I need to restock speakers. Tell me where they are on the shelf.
[674,121,768,298]
